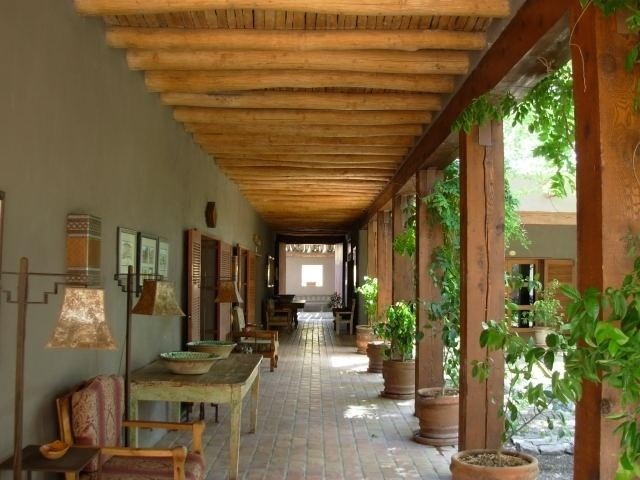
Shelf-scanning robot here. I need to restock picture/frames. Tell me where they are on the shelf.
[140,233,158,292]
[117,227,140,292]
[157,238,171,282]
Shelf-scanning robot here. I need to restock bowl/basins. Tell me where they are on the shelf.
[159,352,223,375]
[39,440,70,460]
[186,340,238,359]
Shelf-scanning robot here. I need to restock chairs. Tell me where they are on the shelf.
[330,298,357,336]
[261,296,292,332]
[231,307,279,372]
[55,370,207,479]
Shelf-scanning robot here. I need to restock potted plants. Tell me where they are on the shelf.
[448,317,596,480]
[378,293,421,400]
[356,276,380,352]
[368,326,392,371]
[413,236,460,445]
[531,298,563,348]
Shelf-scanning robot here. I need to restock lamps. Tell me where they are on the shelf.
[1,212,120,479]
[113,266,188,467]
[197,273,248,423]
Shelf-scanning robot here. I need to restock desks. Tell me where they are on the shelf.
[279,299,306,329]
[3,445,102,479]
[127,347,265,479]
